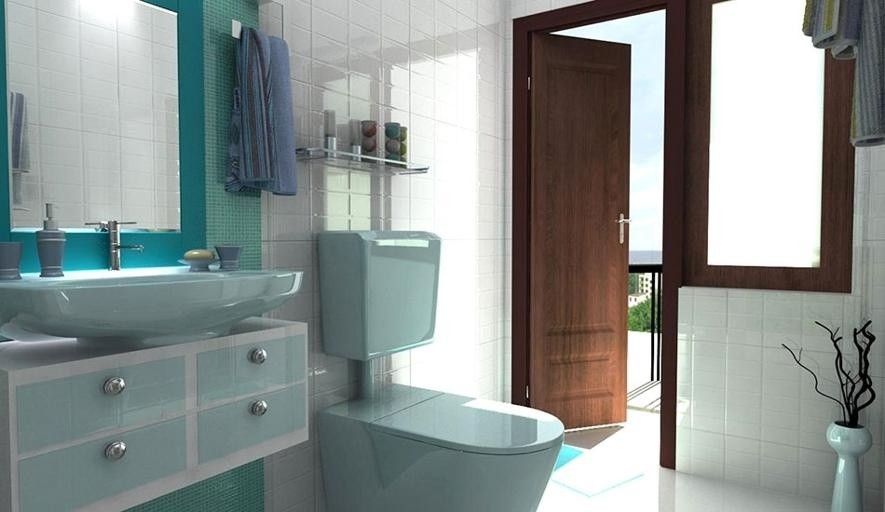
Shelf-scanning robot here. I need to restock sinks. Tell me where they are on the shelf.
[1,266,304,349]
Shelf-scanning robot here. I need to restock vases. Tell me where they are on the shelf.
[824,420,873,512]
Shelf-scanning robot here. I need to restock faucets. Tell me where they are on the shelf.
[85,222,109,234]
[108,220,144,272]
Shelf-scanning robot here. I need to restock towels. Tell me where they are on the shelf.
[800,1,885,148]
[223,26,299,197]
[9,92,30,174]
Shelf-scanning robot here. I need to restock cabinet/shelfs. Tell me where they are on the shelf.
[0,317,308,512]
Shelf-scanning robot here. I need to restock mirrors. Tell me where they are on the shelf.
[4,0,182,234]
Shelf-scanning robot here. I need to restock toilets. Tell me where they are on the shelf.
[316,230,565,512]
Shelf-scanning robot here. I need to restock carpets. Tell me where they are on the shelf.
[552,444,644,496]
